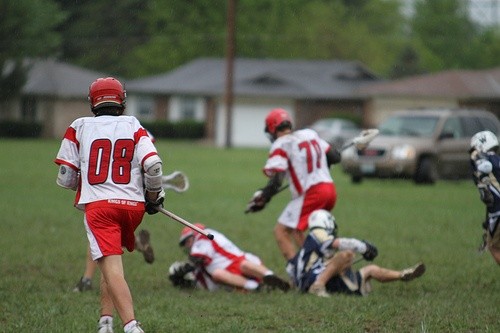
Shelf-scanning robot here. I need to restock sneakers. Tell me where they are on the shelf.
[124,320,145,333]
[96,318,115,333]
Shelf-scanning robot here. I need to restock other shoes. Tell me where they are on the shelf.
[400,262,426,282]
[74,276,93,293]
[254,274,291,294]
[134,230,155,264]
[308,283,329,298]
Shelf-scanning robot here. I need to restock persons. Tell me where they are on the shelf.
[468,130,500,265]
[284,209,425,296]
[250,109,341,280]
[55,77,166,333]
[169,223,291,293]
[71,129,155,292]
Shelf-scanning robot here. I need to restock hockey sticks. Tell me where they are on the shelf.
[160,170,189,192]
[243,128,379,215]
[147,169,214,240]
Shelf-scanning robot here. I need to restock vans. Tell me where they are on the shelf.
[342,108,500,186]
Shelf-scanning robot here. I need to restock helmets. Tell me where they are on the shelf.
[178,223,206,247]
[264,108,292,143]
[87,76,127,112]
[468,130,500,155]
[307,209,336,235]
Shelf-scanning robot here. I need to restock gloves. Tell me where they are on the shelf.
[144,190,166,215]
[248,187,273,213]
[360,239,378,262]
